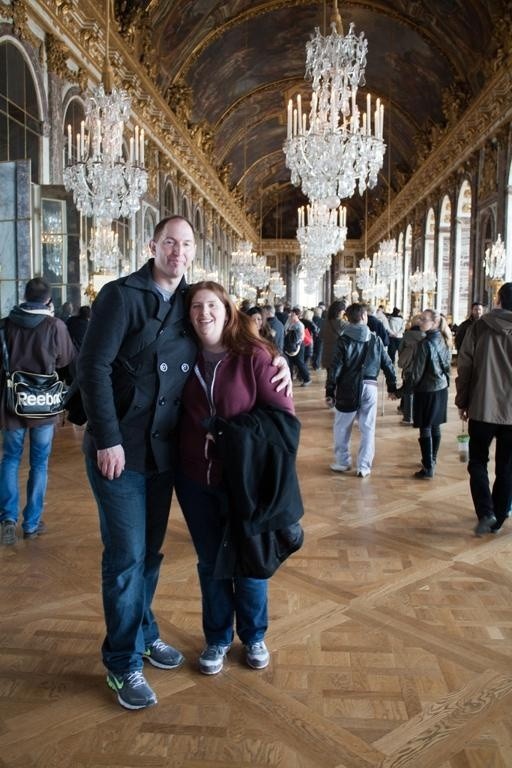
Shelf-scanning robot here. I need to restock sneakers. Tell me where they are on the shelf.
[1,521,17,544]
[106,670,158,710]
[198,642,232,675]
[329,463,351,471]
[142,638,185,670]
[23,521,46,540]
[245,641,270,669]
[357,470,370,477]
[475,514,500,533]
[301,380,312,387]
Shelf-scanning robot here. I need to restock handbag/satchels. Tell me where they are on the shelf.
[5,369,68,419]
[334,362,363,412]
[62,376,87,426]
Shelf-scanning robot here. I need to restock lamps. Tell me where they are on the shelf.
[291,206,348,292]
[406,236,510,299]
[349,209,389,292]
[71,216,227,311]
[282,3,385,208]
[54,0,151,238]
[232,142,286,293]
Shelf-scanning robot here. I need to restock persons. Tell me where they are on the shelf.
[176,280,299,676]
[75,216,294,711]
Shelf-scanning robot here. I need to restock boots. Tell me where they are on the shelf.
[415,436,441,479]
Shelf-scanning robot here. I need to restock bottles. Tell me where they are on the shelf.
[457,434,470,462]
